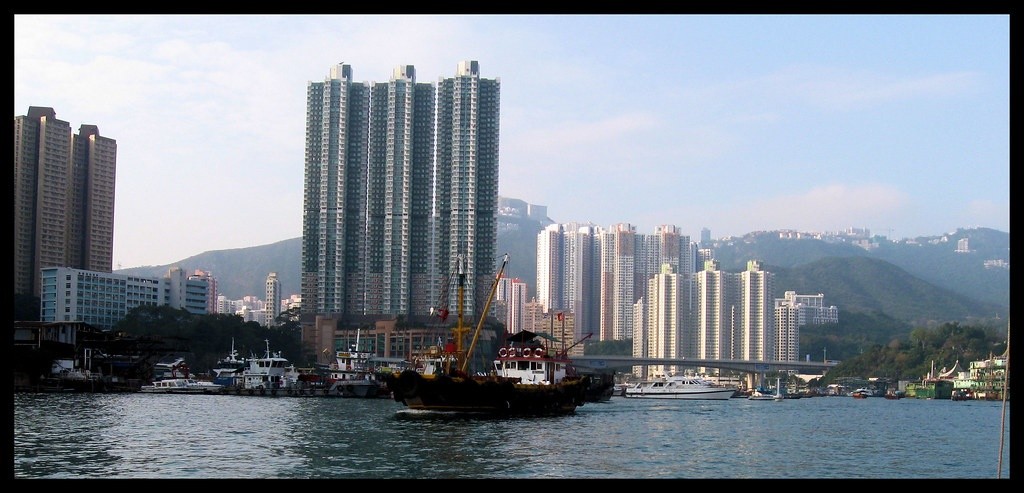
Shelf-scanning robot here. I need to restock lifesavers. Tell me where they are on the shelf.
[508,347,516,357]
[522,348,532,357]
[260,390,265,394]
[310,388,315,394]
[259,385,263,389]
[533,347,544,358]
[249,389,253,394]
[347,385,353,392]
[337,385,344,391]
[396,369,424,399]
[499,348,508,357]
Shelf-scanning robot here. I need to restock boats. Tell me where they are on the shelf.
[382,251,595,419]
[138,378,226,394]
[748,393,776,402]
[624,376,739,400]
[611,384,623,396]
[220,338,381,397]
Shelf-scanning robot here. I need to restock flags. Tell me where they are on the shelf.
[558,312,564,321]
